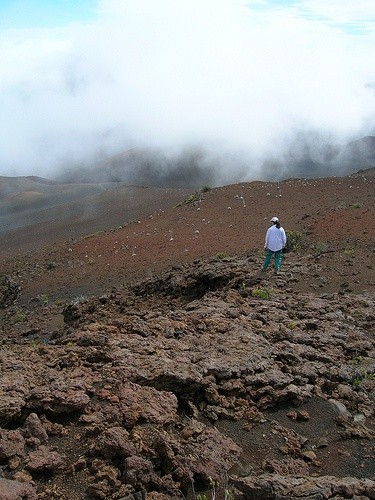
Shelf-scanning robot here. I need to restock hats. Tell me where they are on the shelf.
[270,216,279,223]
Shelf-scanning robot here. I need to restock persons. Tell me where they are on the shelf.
[260,216,287,274]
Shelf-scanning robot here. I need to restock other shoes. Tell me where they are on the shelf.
[262,266,267,271]
[275,270,279,275]
